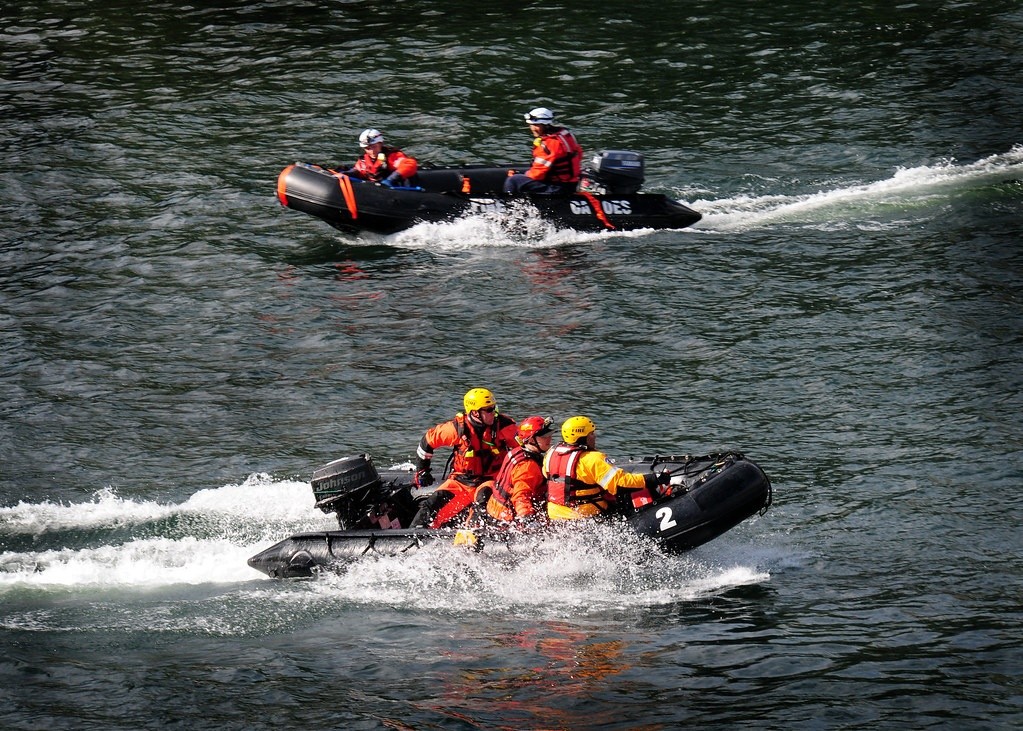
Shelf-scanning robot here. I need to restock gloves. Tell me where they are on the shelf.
[522,514,539,534]
[643,469,671,488]
[415,467,434,489]
[507,170,524,177]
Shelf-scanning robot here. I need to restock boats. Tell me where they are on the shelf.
[247,454,772,581]
[276,151,702,238]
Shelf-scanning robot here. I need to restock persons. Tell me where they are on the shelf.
[503,107,584,205]
[415,388,670,535]
[340,128,418,187]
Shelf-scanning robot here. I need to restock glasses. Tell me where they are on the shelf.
[478,406,495,414]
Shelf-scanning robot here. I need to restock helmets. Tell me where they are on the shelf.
[359,129,384,147]
[562,415,596,445]
[525,107,553,125]
[463,389,496,417]
[518,416,549,447]
